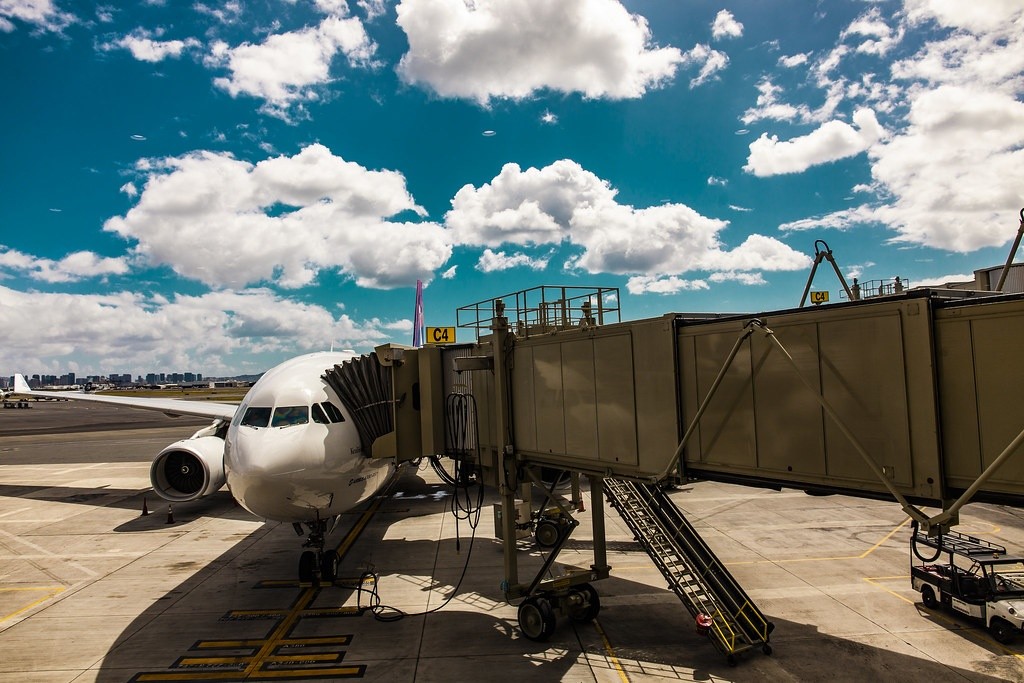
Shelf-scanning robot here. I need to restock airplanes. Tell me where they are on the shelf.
[0,341,414,583]
